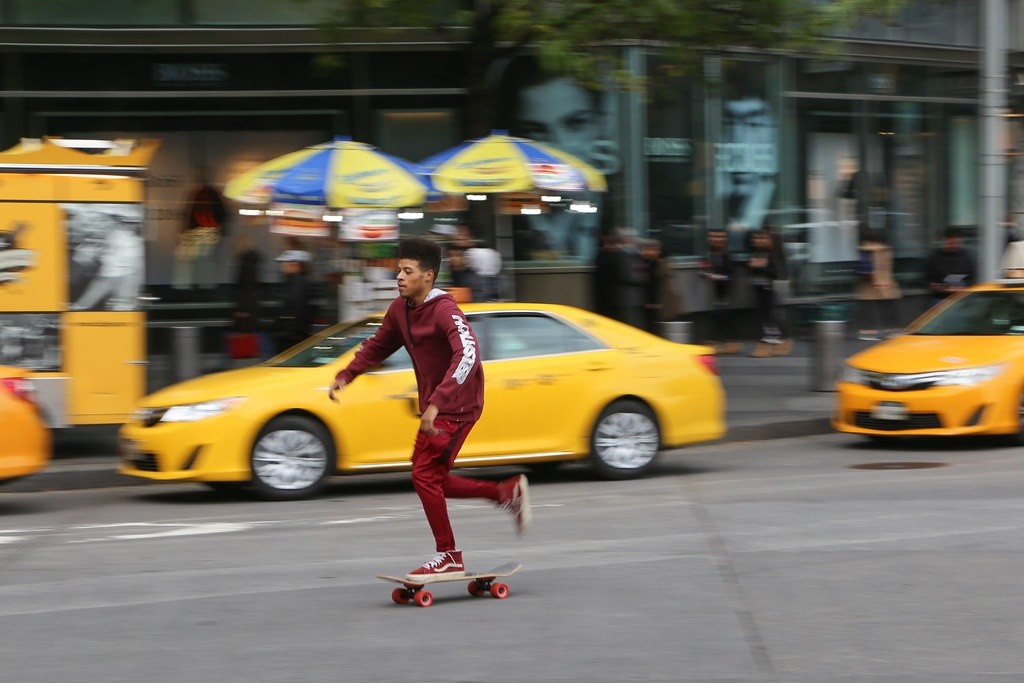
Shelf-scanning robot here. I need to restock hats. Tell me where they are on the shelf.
[275,250,305,263]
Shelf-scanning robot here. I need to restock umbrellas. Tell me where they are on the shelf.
[222,137,444,209]
[420,131,609,197]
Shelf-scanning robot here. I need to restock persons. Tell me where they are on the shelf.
[229,224,976,362]
[329,238,529,582]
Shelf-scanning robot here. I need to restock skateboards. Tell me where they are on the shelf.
[377,561,522,608]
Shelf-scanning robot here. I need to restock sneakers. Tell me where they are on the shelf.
[500,474,530,533]
[405,551,466,582]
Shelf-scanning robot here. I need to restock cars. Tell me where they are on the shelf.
[1,366,54,480]
[831,282,1024,447]
[116,303,727,502]
